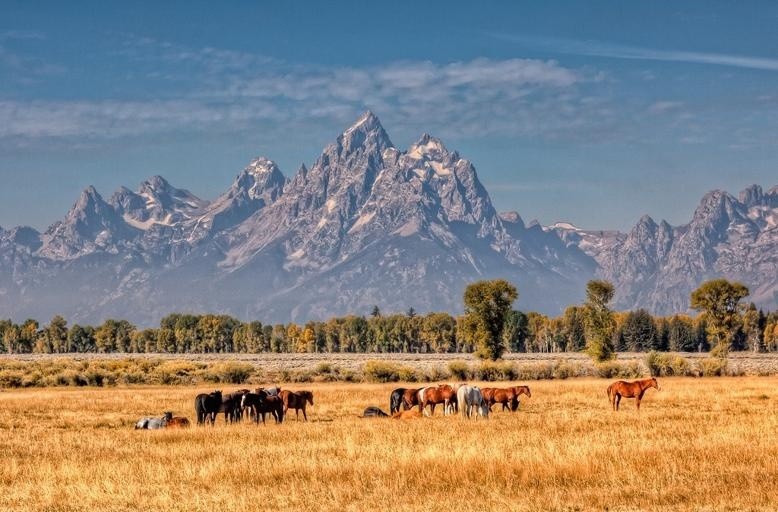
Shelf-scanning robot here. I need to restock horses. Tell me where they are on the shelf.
[165,417,190,429]
[391,384,531,421]
[357,407,390,418]
[195,386,314,427]
[607,377,661,411]
[135,412,172,429]
